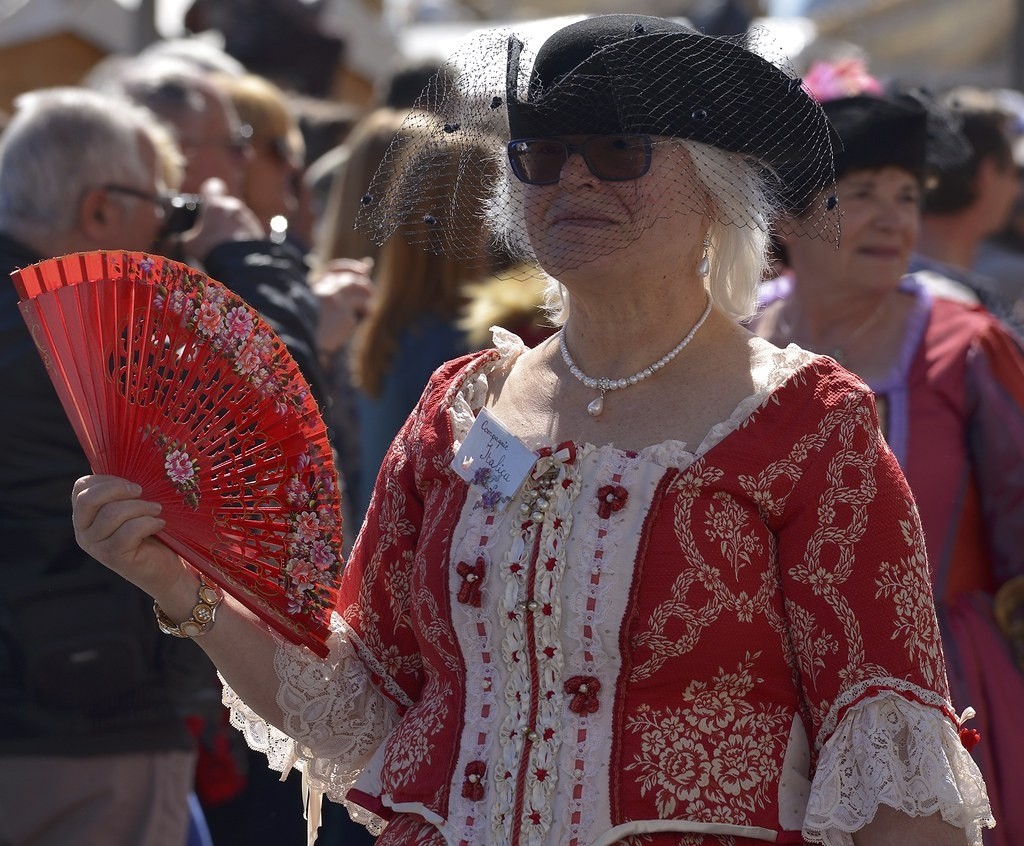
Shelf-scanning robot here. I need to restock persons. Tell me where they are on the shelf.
[0,13,1024,846]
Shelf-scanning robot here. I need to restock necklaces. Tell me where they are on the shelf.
[559,287,713,416]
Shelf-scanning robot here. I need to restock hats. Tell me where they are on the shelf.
[783,93,928,198]
[506,14,848,195]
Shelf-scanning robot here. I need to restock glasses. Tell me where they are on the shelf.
[507,132,684,186]
[102,184,199,231]
[183,134,252,161]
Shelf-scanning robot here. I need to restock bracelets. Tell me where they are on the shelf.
[152,564,224,637]
